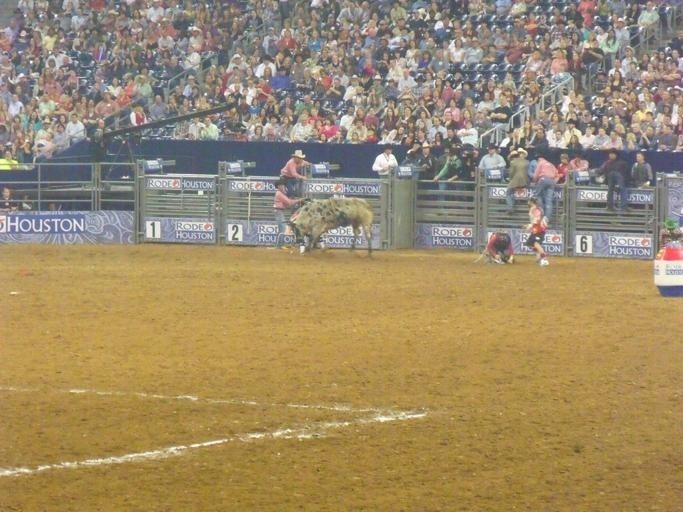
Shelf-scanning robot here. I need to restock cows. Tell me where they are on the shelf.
[279,197,375,257]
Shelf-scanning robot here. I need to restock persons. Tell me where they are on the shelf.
[524,196,550,265]
[274,180,304,250]
[659,218,683,250]
[486,231,513,264]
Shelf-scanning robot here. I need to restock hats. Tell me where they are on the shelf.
[292,150,305,158]
[411,138,432,149]
[486,143,498,150]
[534,151,549,160]
[507,147,528,161]
[606,147,619,156]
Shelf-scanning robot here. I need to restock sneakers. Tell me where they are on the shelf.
[605,206,634,212]
[536,253,550,266]
[279,244,289,250]
